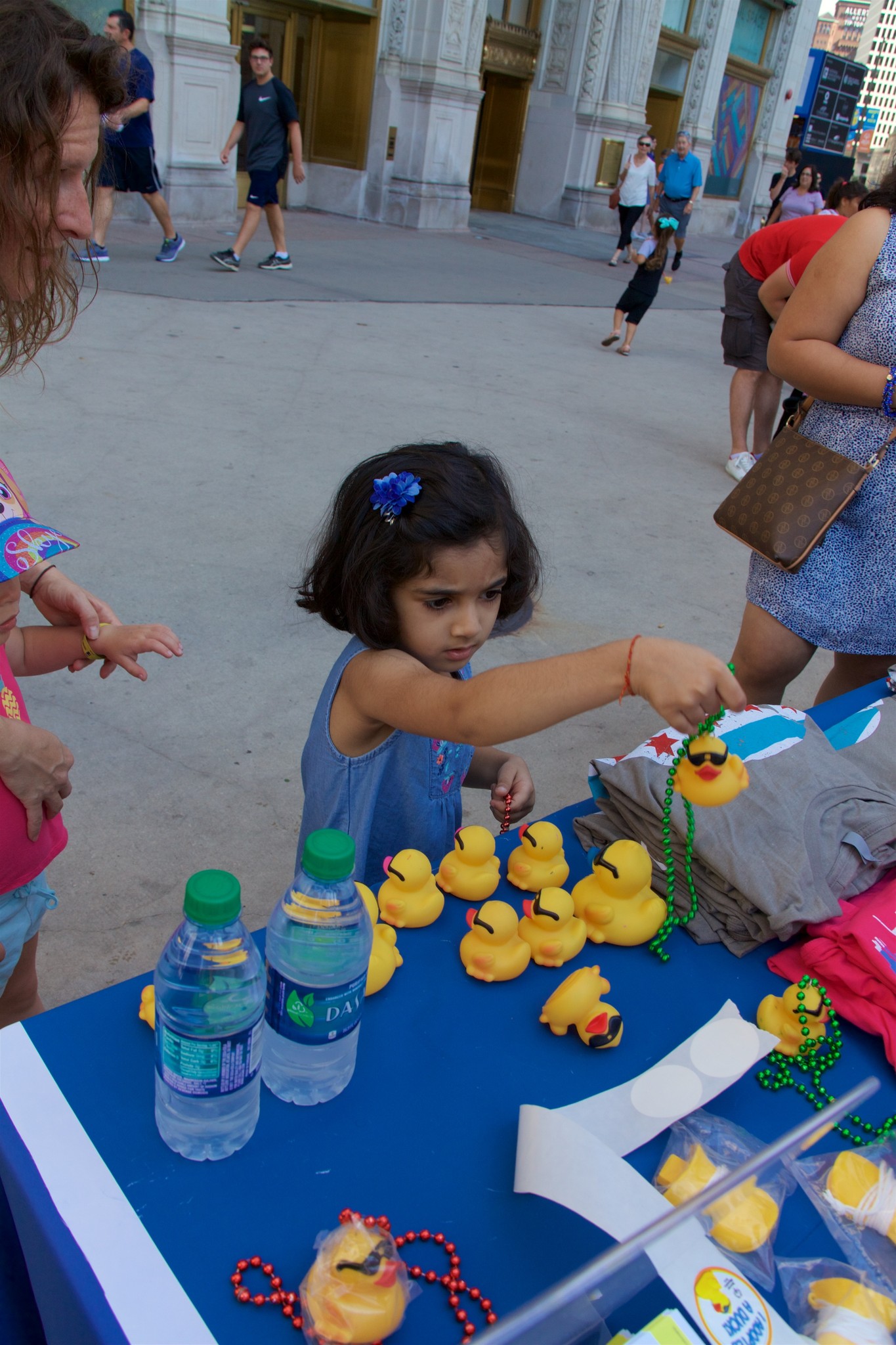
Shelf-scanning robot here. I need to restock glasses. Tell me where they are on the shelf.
[639,140,651,147]
[251,56,270,62]
[801,171,813,177]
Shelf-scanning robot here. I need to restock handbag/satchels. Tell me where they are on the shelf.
[713,410,877,573]
[609,189,620,209]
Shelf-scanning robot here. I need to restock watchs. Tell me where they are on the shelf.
[688,200,694,205]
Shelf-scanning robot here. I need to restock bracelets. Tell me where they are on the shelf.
[648,207,654,210]
[618,635,642,706]
[82,621,113,662]
[656,195,661,199]
[881,366,896,421]
[28,565,57,600]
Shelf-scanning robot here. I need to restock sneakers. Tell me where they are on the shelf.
[259,251,293,270]
[725,450,756,481]
[154,231,186,262]
[72,239,110,262]
[211,248,240,271]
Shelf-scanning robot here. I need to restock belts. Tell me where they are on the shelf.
[663,191,689,204]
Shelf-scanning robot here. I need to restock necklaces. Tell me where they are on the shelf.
[648,661,736,961]
[757,974,896,1148]
[499,792,512,836]
[228,1208,498,1345]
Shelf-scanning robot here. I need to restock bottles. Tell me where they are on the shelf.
[261,828,374,1107]
[151,869,266,1161]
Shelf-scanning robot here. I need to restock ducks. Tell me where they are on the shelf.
[458,899,532,983]
[138,984,156,1032]
[377,848,445,929]
[670,729,750,808]
[756,980,832,1058]
[352,879,404,997]
[570,837,669,947]
[300,1226,406,1344]
[518,886,587,968]
[434,824,502,901]
[505,820,571,893]
[538,963,624,1049]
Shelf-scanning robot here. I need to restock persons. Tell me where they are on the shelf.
[766,149,803,224]
[719,214,851,480]
[765,163,824,227]
[630,134,675,241]
[0,0,126,838]
[288,436,748,894]
[0,492,187,1038]
[654,130,702,272]
[607,135,657,267]
[726,166,895,711]
[71,7,186,262]
[817,178,871,220]
[600,212,679,353]
[209,42,307,273]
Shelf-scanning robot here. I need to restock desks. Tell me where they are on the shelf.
[1,683,896,1345]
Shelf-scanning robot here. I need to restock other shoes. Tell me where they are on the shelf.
[602,330,620,346]
[616,344,630,355]
[671,250,682,270]
[609,259,617,266]
[624,256,632,263]
